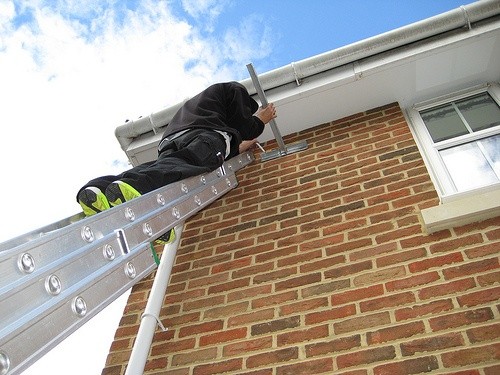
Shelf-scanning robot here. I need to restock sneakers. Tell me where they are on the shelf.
[78,186,111,218]
[106,176,176,245]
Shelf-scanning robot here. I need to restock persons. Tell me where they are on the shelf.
[75,80,278,247]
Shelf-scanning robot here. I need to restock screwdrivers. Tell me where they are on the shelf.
[256,142,267,153]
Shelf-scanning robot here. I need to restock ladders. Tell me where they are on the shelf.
[0,148,256,375]
